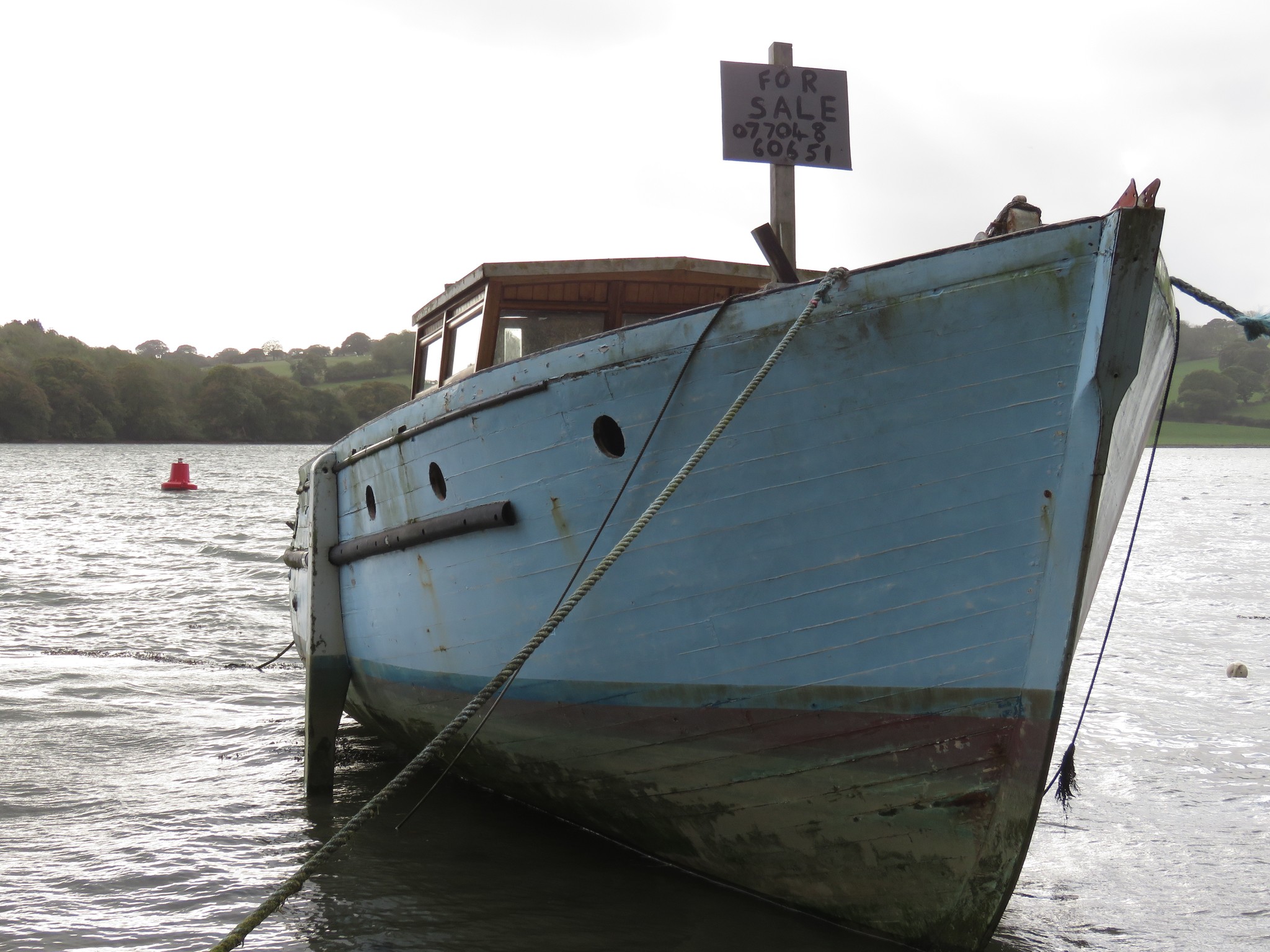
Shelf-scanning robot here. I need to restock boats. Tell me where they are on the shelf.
[284,42,1268,952]
